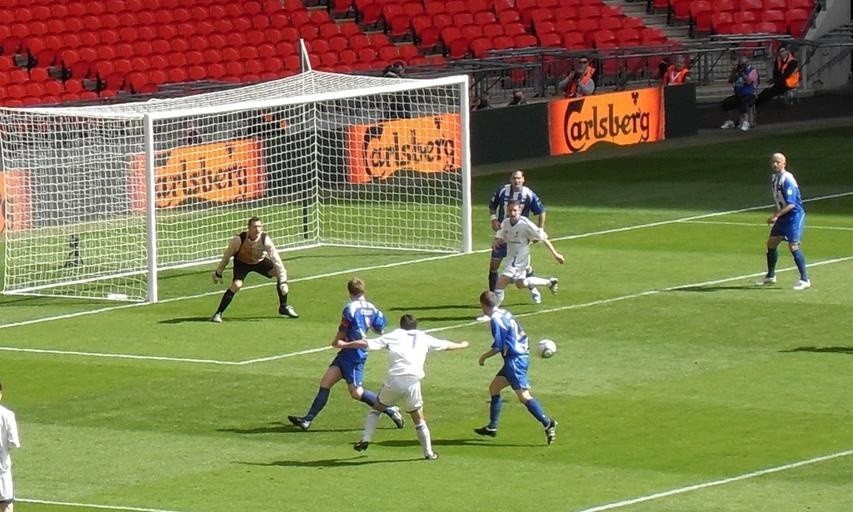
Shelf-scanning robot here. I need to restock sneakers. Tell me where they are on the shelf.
[792,279,811,291]
[719,119,751,133]
[476,314,491,322]
[425,451,439,459]
[754,275,777,286]
[287,415,311,431]
[212,312,222,323]
[474,425,497,437]
[352,440,369,454]
[549,277,559,296]
[390,405,404,428]
[278,304,298,318]
[530,291,541,304]
[543,417,557,446]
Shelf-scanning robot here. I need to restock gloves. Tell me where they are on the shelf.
[210,272,223,285]
[279,283,288,295]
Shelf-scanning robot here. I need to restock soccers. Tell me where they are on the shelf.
[537,339,556,358]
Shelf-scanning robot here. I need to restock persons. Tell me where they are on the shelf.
[330,312,469,460]
[287,275,404,433]
[558,54,596,99]
[754,152,810,291]
[469,90,494,112]
[472,290,558,445]
[475,200,564,324]
[755,44,800,109]
[381,62,412,119]
[661,54,692,86]
[719,56,760,132]
[487,169,546,303]
[0,382,20,512]
[210,216,298,324]
[506,89,527,109]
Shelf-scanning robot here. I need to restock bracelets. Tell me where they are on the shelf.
[278,282,288,285]
[214,270,222,278]
[490,213,496,221]
